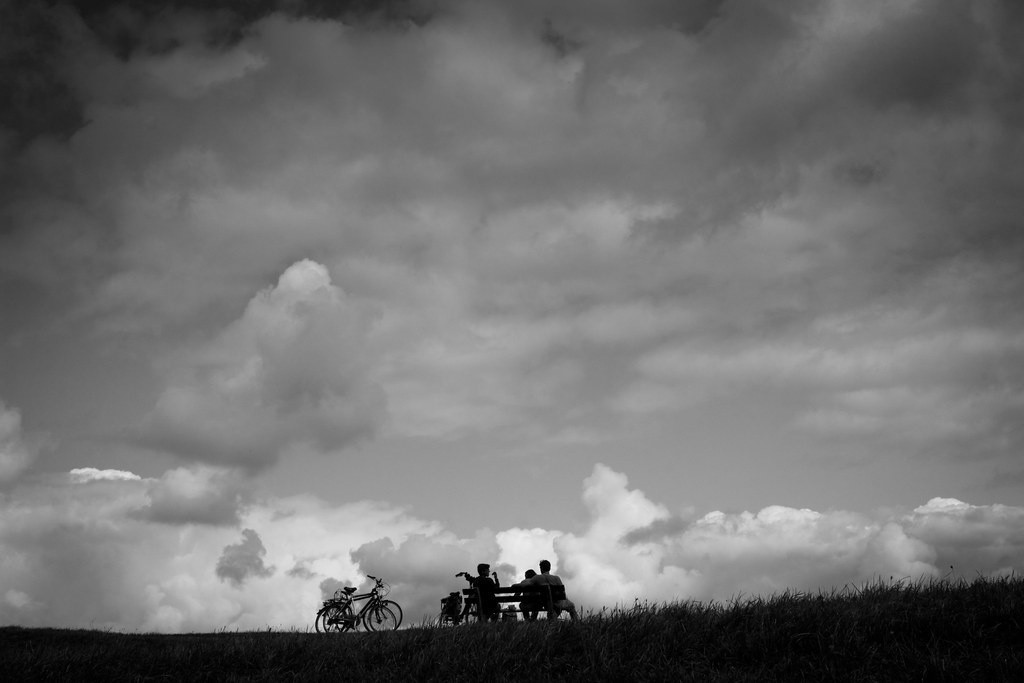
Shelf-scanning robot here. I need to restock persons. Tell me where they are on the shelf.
[464,558,567,622]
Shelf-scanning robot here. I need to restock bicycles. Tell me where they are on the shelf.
[314,574,404,633]
[436,571,498,628]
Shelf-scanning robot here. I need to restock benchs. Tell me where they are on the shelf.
[462,584,567,621]
